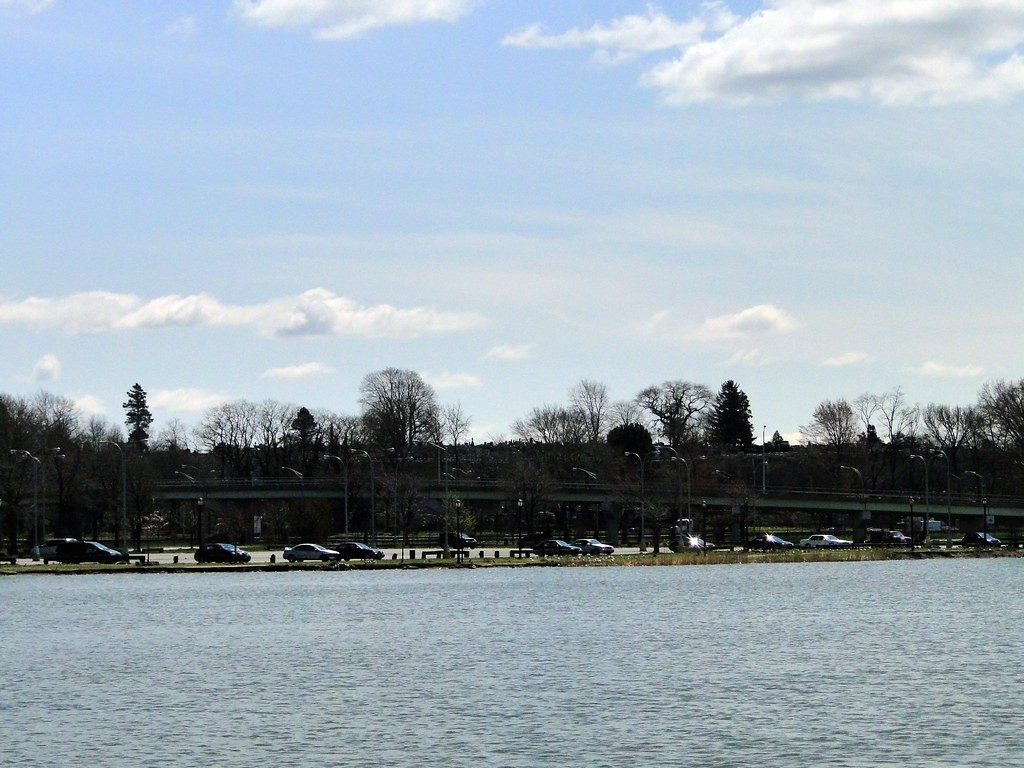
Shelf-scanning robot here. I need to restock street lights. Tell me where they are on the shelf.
[840,465,867,540]
[197,496,203,544]
[351,448,394,548]
[517,498,523,561]
[654,444,685,546]
[762,424,767,496]
[929,448,951,548]
[624,449,661,552]
[393,456,416,545]
[983,497,987,549]
[456,499,461,564]
[910,453,944,548]
[671,455,706,536]
[702,500,708,557]
[964,470,986,548]
[9,447,67,562]
[909,497,914,552]
[323,453,368,542]
[411,438,451,559]
[97,439,149,554]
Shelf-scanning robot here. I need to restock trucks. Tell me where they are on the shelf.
[919,520,942,532]
[669,516,703,535]
[869,528,908,548]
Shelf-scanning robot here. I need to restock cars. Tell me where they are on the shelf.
[667,537,715,553]
[57,538,123,564]
[566,539,615,556]
[30,537,77,558]
[193,542,251,564]
[334,541,385,561]
[533,539,583,557]
[799,534,854,550]
[750,534,794,549]
[283,544,341,562]
[961,531,1002,549]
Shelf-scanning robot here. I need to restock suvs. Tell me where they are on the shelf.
[438,531,478,549]
